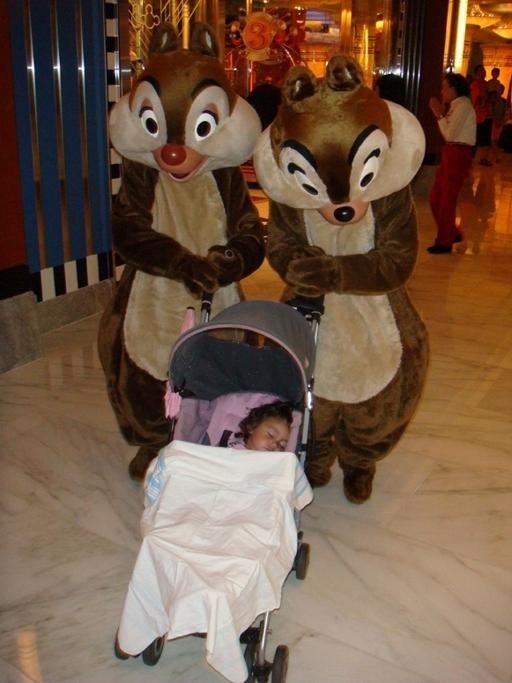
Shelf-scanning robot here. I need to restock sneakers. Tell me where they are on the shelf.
[427,244,452,254]
[454,233,463,243]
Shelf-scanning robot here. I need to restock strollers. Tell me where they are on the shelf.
[113,288,322,681]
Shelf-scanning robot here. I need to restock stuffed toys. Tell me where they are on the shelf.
[251,54,430,504]
[95,21,265,482]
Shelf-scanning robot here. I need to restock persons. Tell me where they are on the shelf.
[470,64,512,165]
[227,399,294,452]
[427,73,478,256]
